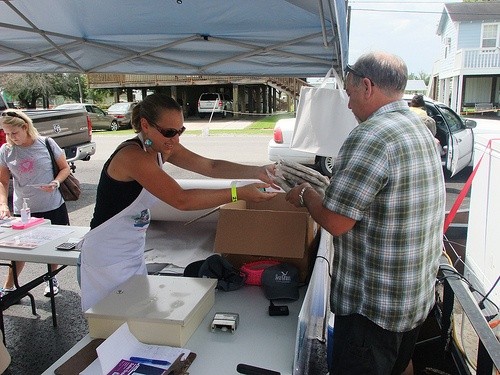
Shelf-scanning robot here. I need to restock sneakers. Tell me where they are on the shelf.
[43,277,60,297]
[0,287,16,298]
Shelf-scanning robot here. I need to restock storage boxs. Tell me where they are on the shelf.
[184,192,314,271]
[85,275,218,347]
[211,312,240,335]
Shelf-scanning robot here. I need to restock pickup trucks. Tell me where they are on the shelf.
[0,90,96,180]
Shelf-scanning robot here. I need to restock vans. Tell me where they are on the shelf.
[198,92,234,118]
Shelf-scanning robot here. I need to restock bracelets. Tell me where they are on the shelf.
[231,185,238,202]
[51,180,60,189]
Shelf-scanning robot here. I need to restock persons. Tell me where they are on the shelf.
[0,108,70,301]
[289,54,446,375]
[78,93,280,311]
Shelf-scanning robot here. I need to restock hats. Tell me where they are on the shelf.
[261,262,300,300]
[412,95,423,104]
[183,254,248,291]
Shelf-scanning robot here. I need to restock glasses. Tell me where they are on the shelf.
[2,112,26,123]
[147,119,186,139]
[343,64,375,87]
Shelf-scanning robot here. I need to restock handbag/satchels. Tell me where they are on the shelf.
[45,136,81,202]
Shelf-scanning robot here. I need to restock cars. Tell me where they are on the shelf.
[105,101,139,129]
[55,103,119,132]
[267,93,477,178]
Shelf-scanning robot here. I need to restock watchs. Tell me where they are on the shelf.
[298,184,313,207]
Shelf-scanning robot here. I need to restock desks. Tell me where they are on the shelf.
[0,219,306,375]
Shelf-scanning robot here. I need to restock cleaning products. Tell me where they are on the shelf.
[20,196,31,221]
